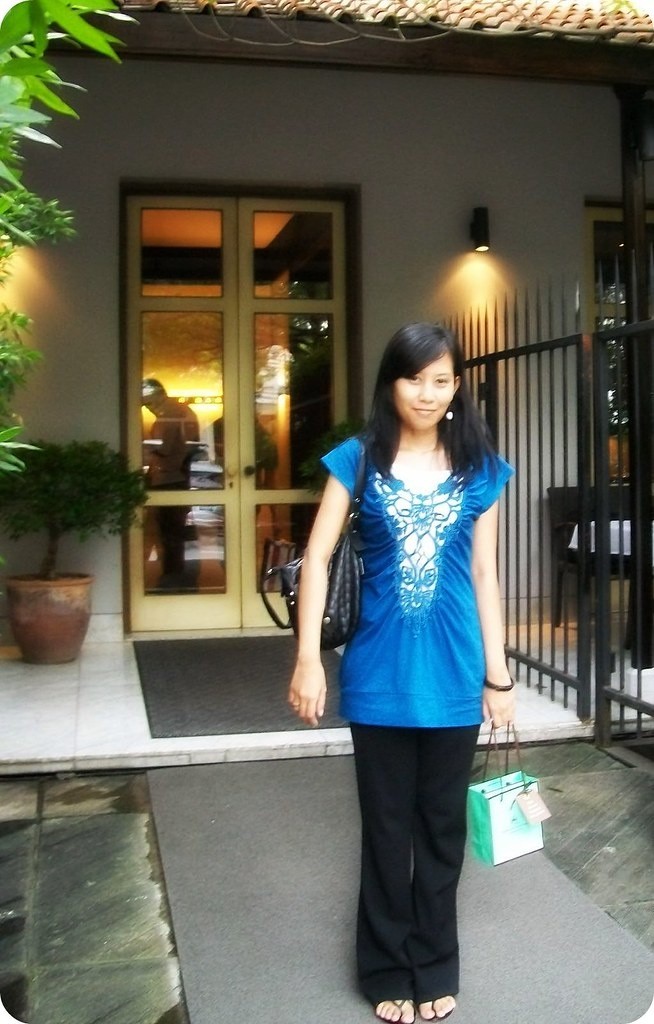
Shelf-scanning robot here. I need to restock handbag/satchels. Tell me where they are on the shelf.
[466,717,553,866]
[263,435,369,652]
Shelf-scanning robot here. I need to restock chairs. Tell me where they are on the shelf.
[547,486,635,654]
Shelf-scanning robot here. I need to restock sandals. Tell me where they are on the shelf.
[418,995,457,1022]
[375,998,418,1024]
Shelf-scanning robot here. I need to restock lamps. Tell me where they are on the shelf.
[469,205,490,252]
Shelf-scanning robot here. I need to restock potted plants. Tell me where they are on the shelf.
[0,440,152,664]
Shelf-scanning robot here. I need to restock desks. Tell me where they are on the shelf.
[567,517,653,650]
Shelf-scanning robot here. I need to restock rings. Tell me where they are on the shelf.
[291,703,300,707]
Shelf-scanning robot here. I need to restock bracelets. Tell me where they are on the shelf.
[484,676,514,691]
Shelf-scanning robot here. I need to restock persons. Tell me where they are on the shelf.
[141,379,200,595]
[287,322,516,1024]
